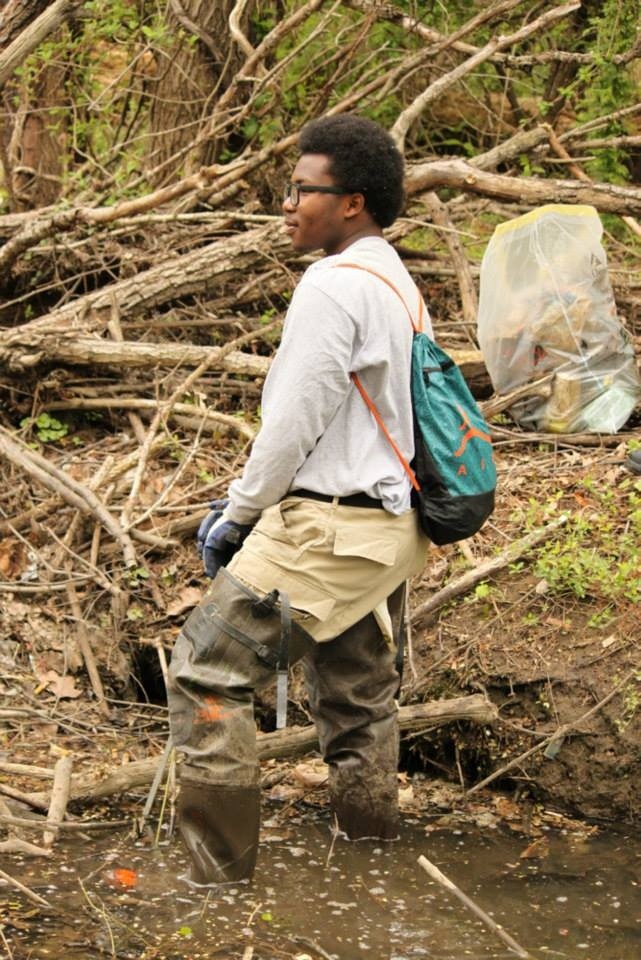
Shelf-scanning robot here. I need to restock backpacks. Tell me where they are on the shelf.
[328,265,496,545]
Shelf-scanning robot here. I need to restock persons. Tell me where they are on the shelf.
[108,115,438,891]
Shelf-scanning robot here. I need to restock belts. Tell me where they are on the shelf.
[288,488,384,509]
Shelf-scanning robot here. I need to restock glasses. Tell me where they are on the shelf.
[283,183,363,207]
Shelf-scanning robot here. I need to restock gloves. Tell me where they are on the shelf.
[198,500,255,579]
